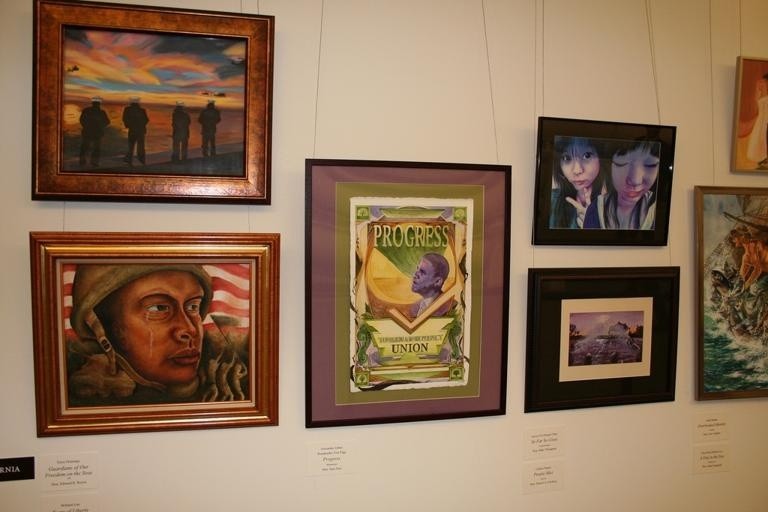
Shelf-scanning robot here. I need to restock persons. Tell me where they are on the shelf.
[67,262,248,404]
[550,143,607,230]
[583,140,660,230]
[408,253,457,318]
[77,94,223,166]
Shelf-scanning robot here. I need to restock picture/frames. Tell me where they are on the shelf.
[524,266,680,412]
[694,185,768,400]
[29,231,280,438]
[304,158,512,428]
[531,116,677,246]
[730,56,768,175]
[32,0,276,203]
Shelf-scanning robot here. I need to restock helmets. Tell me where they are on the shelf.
[70,264,213,341]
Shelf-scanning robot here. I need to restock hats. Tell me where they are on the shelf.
[176,100,185,108]
[208,100,215,105]
[128,96,140,104]
[91,95,103,103]
